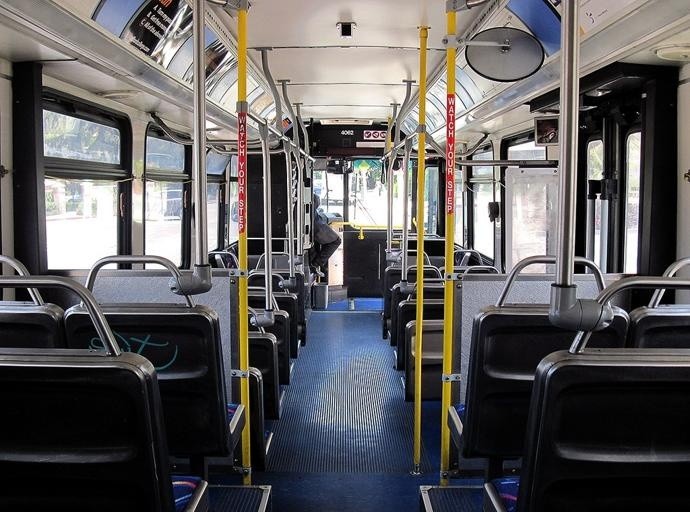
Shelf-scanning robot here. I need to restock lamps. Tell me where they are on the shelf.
[655,44,690,62]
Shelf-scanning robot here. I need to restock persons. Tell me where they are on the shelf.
[314,195,320,209]
[291,152,342,277]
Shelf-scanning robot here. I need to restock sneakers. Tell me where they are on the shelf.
[310,263,325,278]
